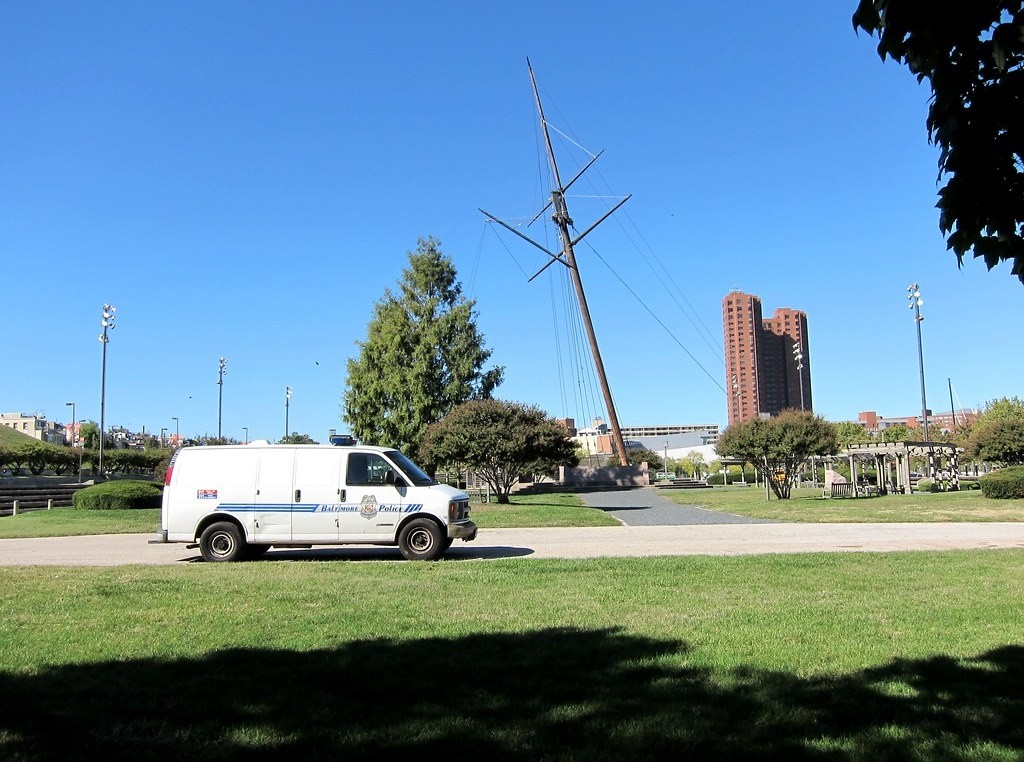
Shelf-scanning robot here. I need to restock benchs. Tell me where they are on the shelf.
[823,482,853,498]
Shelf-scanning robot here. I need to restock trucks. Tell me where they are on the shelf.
[147,435,478,563]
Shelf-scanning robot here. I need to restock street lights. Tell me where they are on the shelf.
[243,427,248,445]
[216,356,228,439]
[99,302,117,476]
[172,417,178,443]
[160,428,167,449]
[907,283,928,443]
[285,386,293,437]
[66,402,75,447]
[78,420,85,484]
